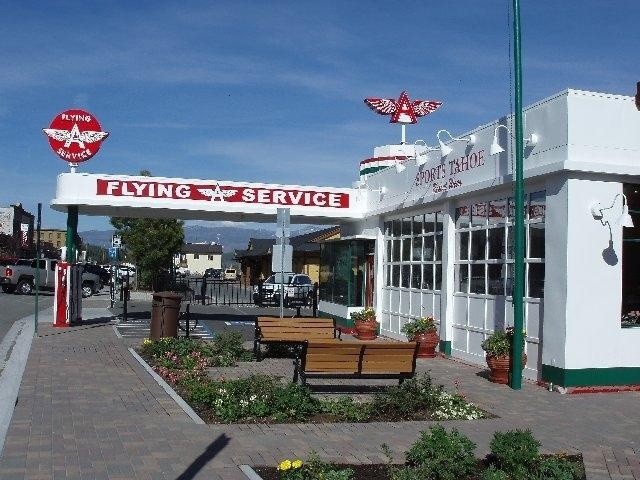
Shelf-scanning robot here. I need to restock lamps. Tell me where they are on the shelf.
[394,155,415,173]
[412,139,440,166]
[593,193,634,229]
[436,129,476,156]
[489,124,537,155]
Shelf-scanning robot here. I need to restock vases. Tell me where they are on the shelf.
[356,319,377,339]
[486,352,527,384]
[411,332,440,358]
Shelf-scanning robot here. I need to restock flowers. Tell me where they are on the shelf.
[401,315,438,340]
[482,327,527,356]
[349,307,376,320]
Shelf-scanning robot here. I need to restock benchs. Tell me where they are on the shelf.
[292,340,421,391]
[252,316,342,361]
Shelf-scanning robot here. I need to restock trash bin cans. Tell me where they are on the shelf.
[151,291,184,340]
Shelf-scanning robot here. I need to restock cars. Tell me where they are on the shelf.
[168,263,186,278]
[0,257,136,298]
[252,273,315,309]
[205,268,241,282]
